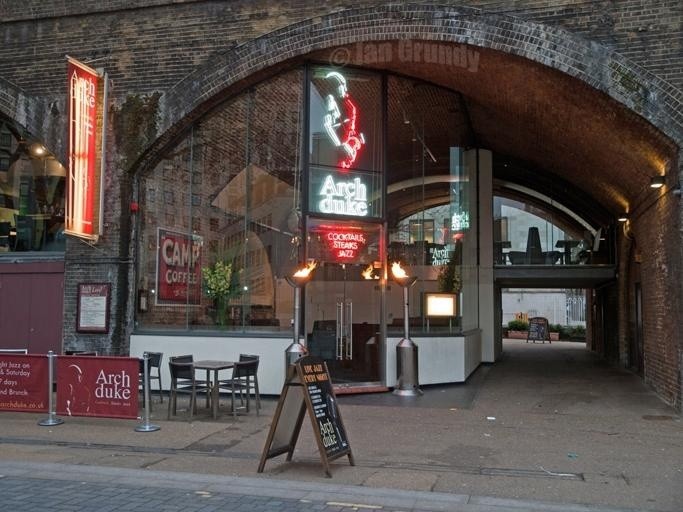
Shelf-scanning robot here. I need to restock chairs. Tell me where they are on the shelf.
[138,350,262,421]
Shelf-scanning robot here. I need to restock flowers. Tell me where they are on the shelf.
[202,260,244,325]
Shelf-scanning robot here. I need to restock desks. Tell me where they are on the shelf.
[555,240,580,264]
[493,241,511,264]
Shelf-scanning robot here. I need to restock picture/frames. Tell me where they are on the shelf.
[78,282,112,333]
[138,289,149,313]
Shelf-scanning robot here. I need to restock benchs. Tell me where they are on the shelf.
[578,227,607,264]
[508,227,561,264]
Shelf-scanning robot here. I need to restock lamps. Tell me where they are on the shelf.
[650,175,665,188]
[618,212,629,221]
